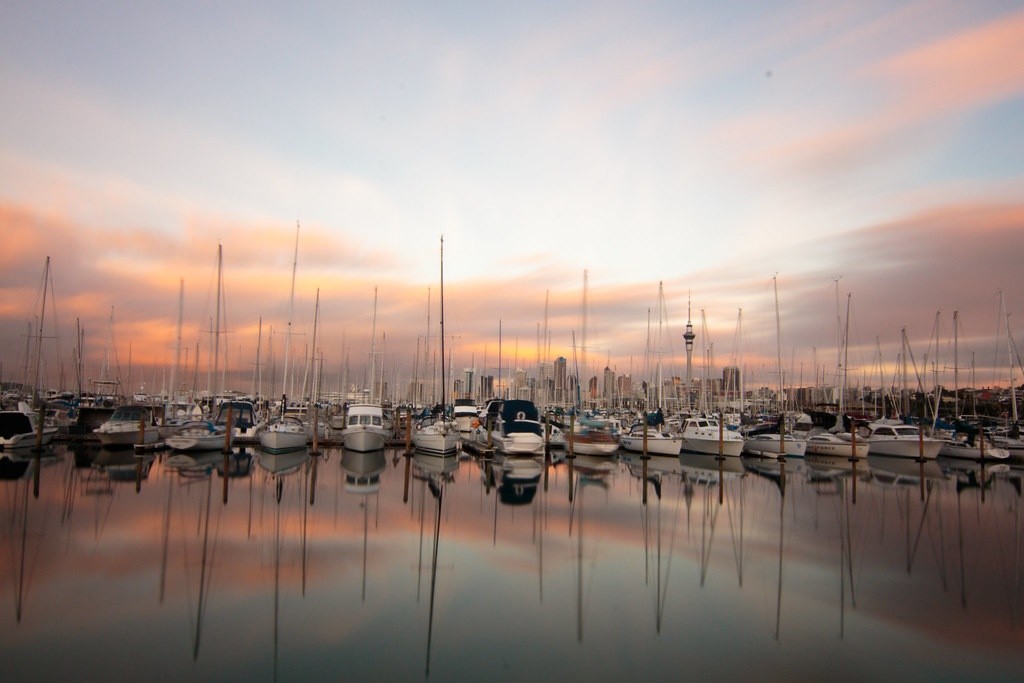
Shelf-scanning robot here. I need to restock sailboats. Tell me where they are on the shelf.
[0,219,1024,498]
